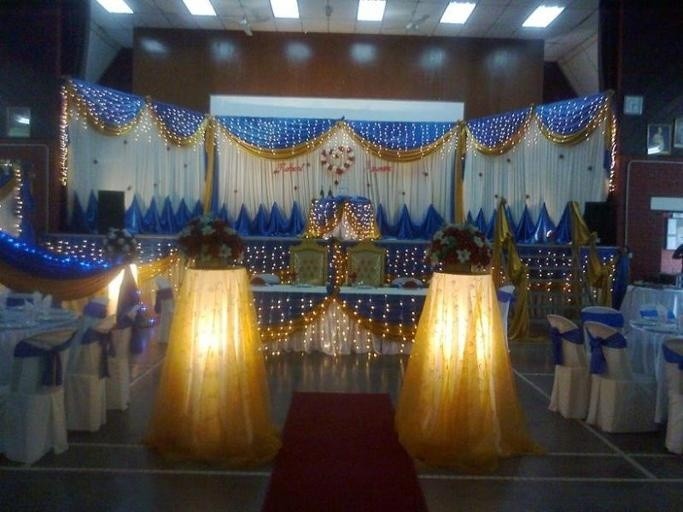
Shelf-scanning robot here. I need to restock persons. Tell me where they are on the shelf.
[652,127,665,151]
[673,244,683,259]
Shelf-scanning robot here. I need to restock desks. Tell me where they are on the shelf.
[247,284,426,370]
[620,284,683,313]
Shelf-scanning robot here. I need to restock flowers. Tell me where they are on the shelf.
[426,223,492,268]
[96,227,139,262]
[174,216,243,262]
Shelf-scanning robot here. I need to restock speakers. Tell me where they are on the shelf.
[585,202,616,246]
[98,190,124,235]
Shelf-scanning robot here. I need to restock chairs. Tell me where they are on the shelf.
[0,278,173,465]
[287,240,386,286]
[495,285,516,349]
[547,301,683,454]
